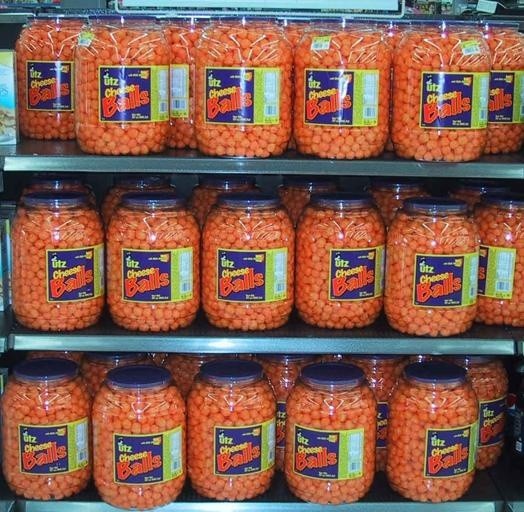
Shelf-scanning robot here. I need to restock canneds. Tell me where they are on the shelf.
[106,190,201,334]
[277,175,338,226]
[1,357,91,500]
[190,175,262,231]
[201,193,294,332]
[457,357,509,471]
[385,361,480,503]
[162,352,229,401]
[15,15,86,140]
[294,192,385,329]
[16,171,97,206]
[72,13,170,157]
[341,353,402,471]
[186,359,277,501]
[480,20,524,154]
[384,197,479,338]
[80,353,155,402]
[294,18,391,160]
[90,363,186,511]
[100,173,175,226]
[470,191,524,328]
[254,353,314,472]
[391,18,491,162]
[10,192,105,331]
[195,15,293,158]
[284,363,378,506]
[159,14,209,150]
[364,178,422,231]
[448,179,486,212]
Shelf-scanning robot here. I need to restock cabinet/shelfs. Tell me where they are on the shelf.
[1,3,524,507]
